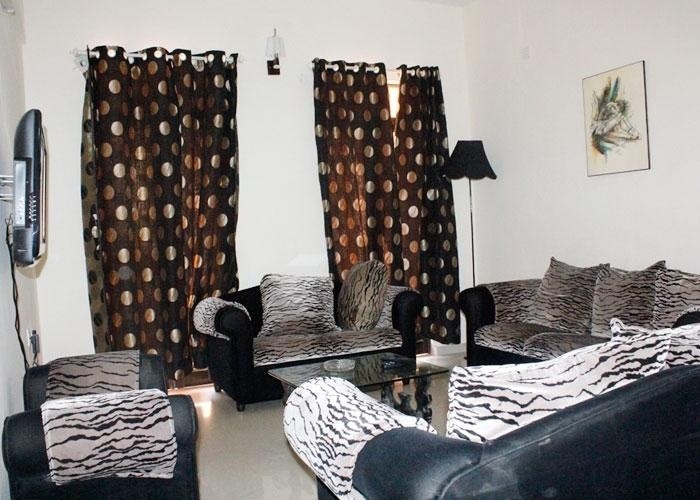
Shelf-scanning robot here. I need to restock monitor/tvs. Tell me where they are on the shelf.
[14,108,47,272]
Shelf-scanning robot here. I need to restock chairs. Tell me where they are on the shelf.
[2,348,201,499]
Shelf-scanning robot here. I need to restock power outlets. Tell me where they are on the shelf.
[26,328,33,347]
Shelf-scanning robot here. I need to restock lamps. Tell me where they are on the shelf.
[441,139,497,289]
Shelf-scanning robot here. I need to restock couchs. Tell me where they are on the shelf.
[454,275,697,366]
[283,365,699,500]
[188,283,423,412]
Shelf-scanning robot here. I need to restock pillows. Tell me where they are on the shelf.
[523,256,608,335]
[590,259,668,337]
[446,329,671,442]
[651,268,699,328]
[605,313,700,367]
[258,271,340,334]
[335,260,390,331]
